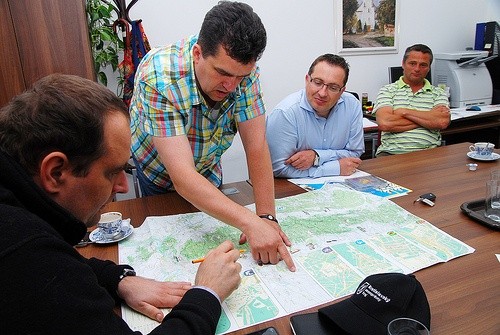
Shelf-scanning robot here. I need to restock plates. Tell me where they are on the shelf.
[467,151,500,161]
[88,223,134,244]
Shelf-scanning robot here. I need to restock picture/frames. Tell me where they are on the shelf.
[333,0,401,56]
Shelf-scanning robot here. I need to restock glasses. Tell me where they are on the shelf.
[309,73,346,93]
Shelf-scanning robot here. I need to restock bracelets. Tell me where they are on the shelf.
[313,150,319,166]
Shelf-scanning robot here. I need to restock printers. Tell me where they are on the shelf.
[430,50,498,108]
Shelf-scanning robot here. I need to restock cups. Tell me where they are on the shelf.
[97,211,122,239]
[484,170,500,224]
[468,141,495,159]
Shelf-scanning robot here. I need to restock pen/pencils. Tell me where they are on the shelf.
[192,249,246,264]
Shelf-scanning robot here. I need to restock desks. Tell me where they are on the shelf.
[363,109,500,137]
[74,142,500,335]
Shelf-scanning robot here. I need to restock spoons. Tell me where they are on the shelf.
[75,239,89,248]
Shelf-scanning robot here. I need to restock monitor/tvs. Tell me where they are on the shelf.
[388,67,432,85]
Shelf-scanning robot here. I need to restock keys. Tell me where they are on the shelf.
[420,193,436,204]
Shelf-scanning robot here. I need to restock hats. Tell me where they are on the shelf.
[318,274,430,335]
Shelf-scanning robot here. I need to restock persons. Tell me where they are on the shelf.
[266,54,365,178]
[372,44,451,158]
[129,0,296,272]
[0,72,243,335]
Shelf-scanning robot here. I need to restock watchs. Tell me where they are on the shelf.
[119,269,136,280]
[259,214,278,223]
